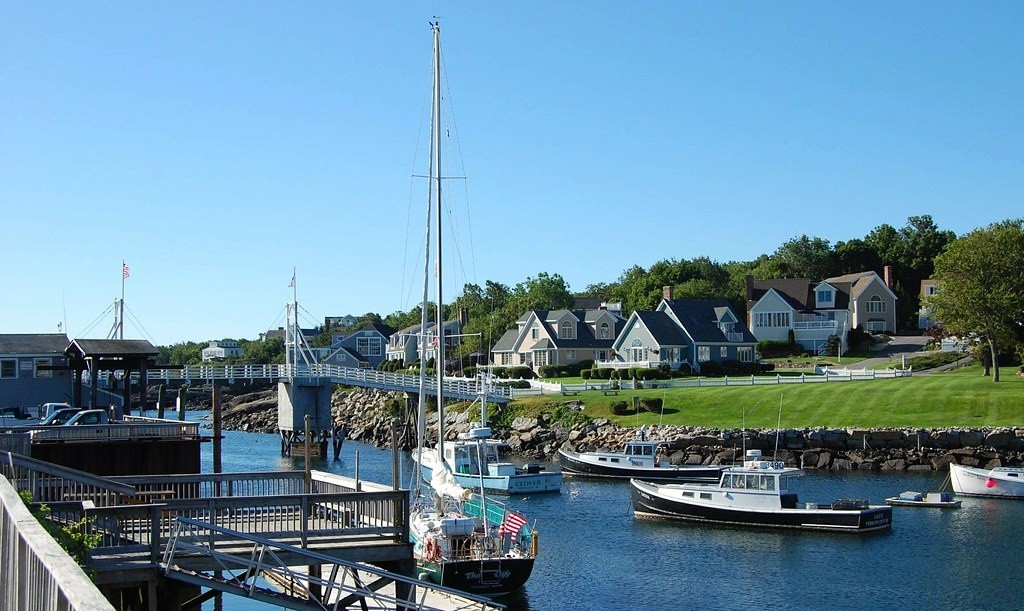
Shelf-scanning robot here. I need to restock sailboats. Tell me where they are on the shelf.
[394,14,565,598]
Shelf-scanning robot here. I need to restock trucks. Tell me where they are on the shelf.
[0,403,166,438]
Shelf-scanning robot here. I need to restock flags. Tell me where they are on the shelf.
[498,511,527,543]
[123,264,129,279]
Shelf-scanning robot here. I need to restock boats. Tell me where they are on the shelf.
[887,495,963,509]
[550,390,741,481]
[629,390,894,534]
[950,461,1024,499]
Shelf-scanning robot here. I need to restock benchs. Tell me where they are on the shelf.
[601,390,620,396]
[645,382,671,389]
[314,503,354,528]
[560,390,581,396]
[64,490,176,518]
